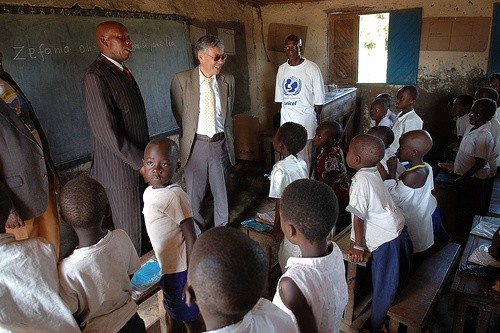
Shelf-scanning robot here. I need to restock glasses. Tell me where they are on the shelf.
[206,54,227,61]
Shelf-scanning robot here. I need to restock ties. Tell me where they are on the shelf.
[204,78,216,138]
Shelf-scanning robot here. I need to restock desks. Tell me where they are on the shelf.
[132,88,500,333]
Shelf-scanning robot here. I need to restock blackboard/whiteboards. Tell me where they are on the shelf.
[0,5,197,169]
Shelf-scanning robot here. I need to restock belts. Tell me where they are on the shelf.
[195,132,225,142]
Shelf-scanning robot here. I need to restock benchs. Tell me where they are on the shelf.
[388,242,461,333]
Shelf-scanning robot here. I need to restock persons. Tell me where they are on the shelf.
[81,21,149,259]
[0,183,83,333]
[435,73,500,225]
[313,121,350,205]
[368,85,423,177]
[56,174,147,333]
[142,138,205,333]
[269,122,335,275]
[0,52,61,264]
[275,34,325,167]
[346,134,414,333]
[273,179,349,333]
[173,34,235,227]
[384,130,437,252]
[188,226,297,333]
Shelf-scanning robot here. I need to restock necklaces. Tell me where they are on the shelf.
[405,164,424,171]
